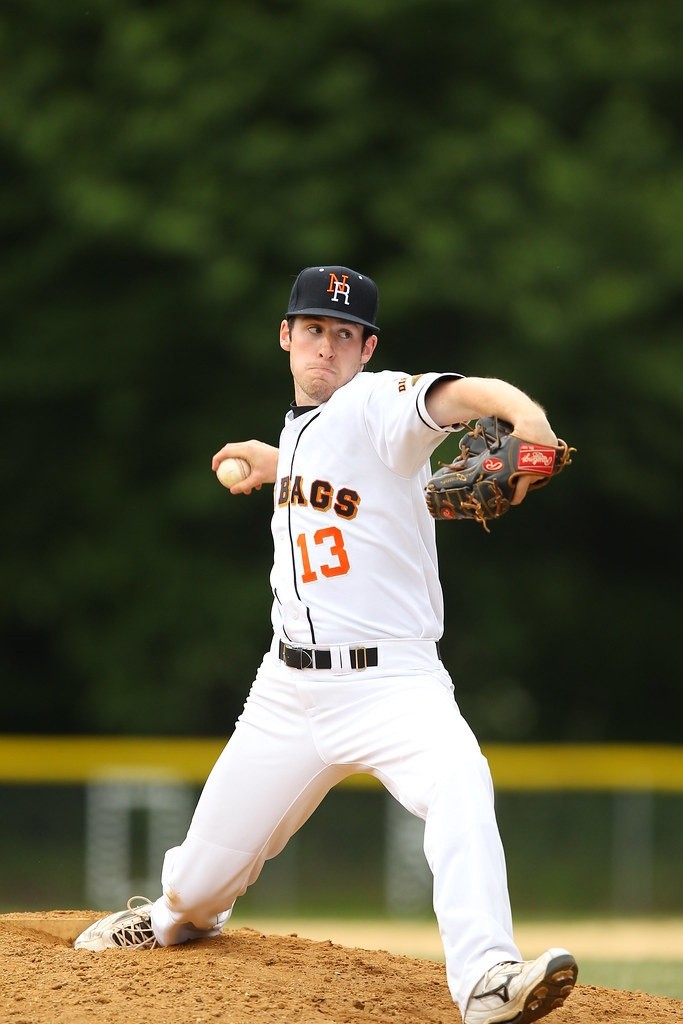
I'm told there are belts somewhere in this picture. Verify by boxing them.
[279,641,378,670]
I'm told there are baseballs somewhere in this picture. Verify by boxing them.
[216,457,251,488]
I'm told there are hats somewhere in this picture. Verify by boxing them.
[285,266,380,331]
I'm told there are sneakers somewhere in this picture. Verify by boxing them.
[464,948,579,1024]
[73,896,162,952]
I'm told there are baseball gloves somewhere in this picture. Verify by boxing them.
[420,397,578,529]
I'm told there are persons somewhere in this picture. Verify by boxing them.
[75,266,580,1023]
